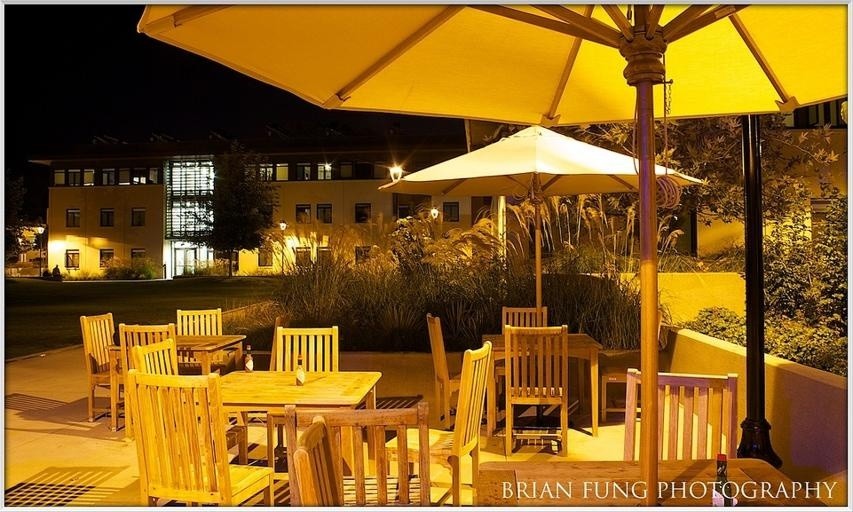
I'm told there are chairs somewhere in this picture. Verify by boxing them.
[78,305,738,507]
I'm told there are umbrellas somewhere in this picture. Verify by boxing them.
[377,124,711,428]
[135,4,848,507]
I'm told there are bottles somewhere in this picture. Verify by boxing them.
[711,453,734,507]
[244,344,254,373]
[294,355,305,386]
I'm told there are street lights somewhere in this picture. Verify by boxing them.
[35,223,44,277]
[388,162,401,229]
[278,219,287,275]
[429,208,438,241]
